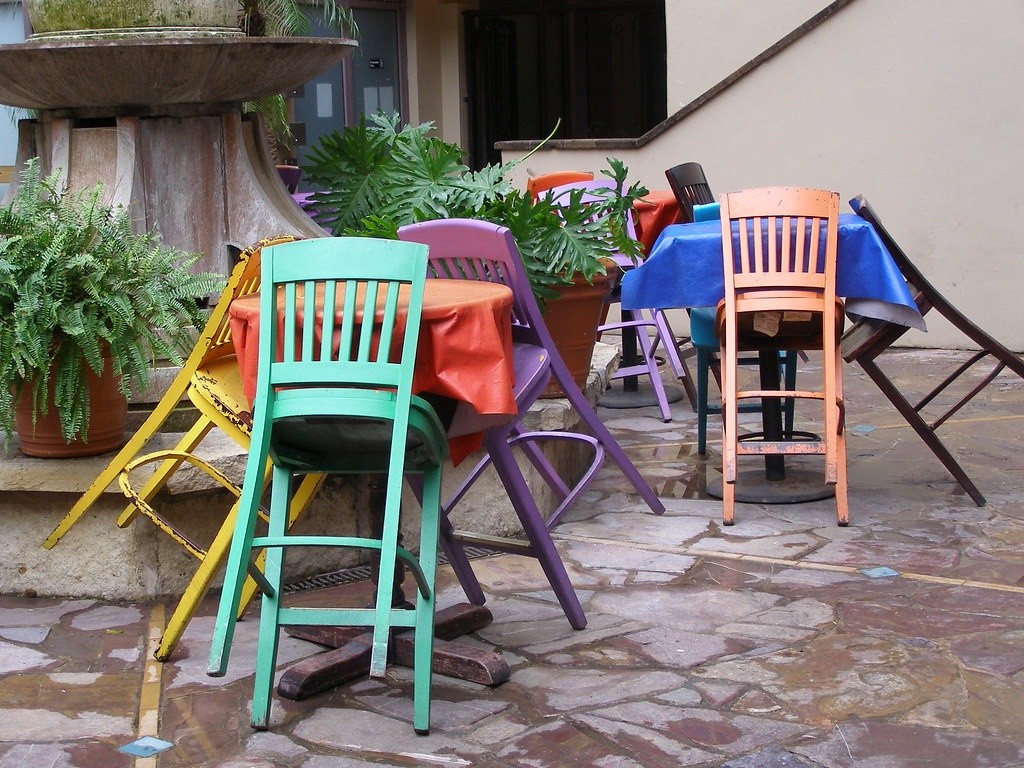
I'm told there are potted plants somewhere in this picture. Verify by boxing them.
[318,111,648,398]
[0,146,226,459]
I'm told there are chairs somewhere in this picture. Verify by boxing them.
[41,163,1024,739]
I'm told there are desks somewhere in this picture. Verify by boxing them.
[618,213,930,505]
[227,278,519,703]
[596,190,694,409]
